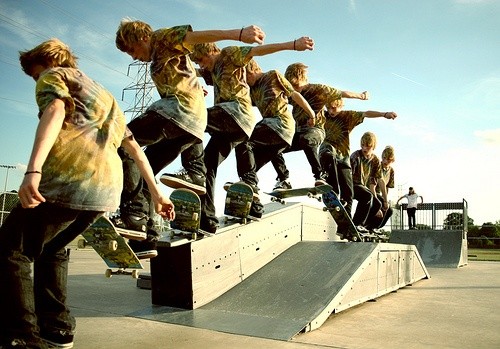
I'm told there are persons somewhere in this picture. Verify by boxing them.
[348,131,390,236]
[270,63,370,188]
[106,17,266,240]
[367,145,396,237]
[158,35,314,237]
[224,57,317,221]
[0,37,178,349]
[314,98,397,238]
[397,183,424,229]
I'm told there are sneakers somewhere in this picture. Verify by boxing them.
[160,169,207,196]
[224,181,259,202]
[314,178,329,187]
[198,218,217,237]
[128,236,158,259]
[40,328,74,348]
[108,210,149,241]
[372,228,383,235]
[355,225,370,235]
[247,204,263,221]
[273,181,292,190]
[336,227,342,236]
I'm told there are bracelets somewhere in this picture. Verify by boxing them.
[239,28,244,41]
[294,39,297,50]
[24,171,44,176]
[363,92,367,100]
[384,112,388,117]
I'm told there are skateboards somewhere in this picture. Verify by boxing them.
[223,182,254,224]
[263,184,333,205]
[167,187,202,240]
[360,232,377,242]
[322,190,363,241]
[378,234,390,243]
[78,215,144,279]
